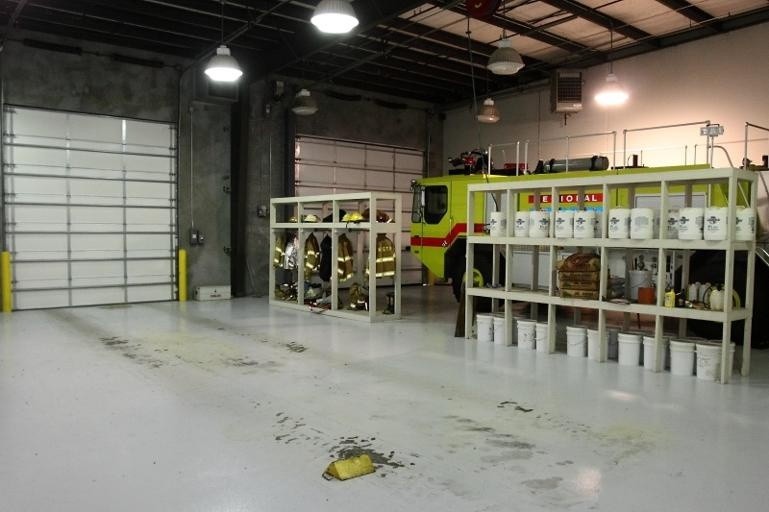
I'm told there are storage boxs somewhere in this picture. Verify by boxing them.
[194,283,233,301]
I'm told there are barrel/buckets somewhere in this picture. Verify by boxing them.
[475,311,549,353]
[606,325,622,360]
[627,269,653,301]
[682,336,707,374]
[575,321,597,357]
[642,335,668,373]
[565,324,586,358]
[668,338,694,376]
[586,327,610,364]
[707,339,736,377]
[653,331,677,369]
[489,206,757,242]
[616,331,641,367]
[627,329,652,366]
[694,343,720,381]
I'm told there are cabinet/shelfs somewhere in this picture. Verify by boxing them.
[463,168,758,386]
[267,191,403,325]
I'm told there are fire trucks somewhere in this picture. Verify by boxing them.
[408,139,767,346]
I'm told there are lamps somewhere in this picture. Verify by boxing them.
[484,2,528,76]
[289,53,316,118]
[591,23,637,109]
[308,2,364,36]
[474,69,503,126]
[204,1,247,84]
